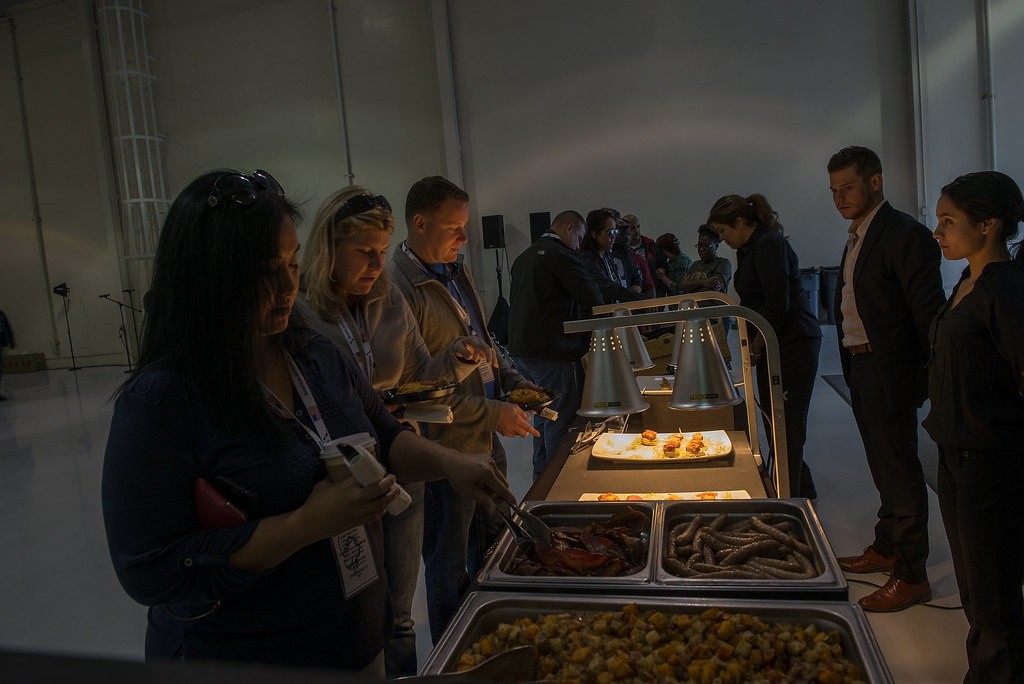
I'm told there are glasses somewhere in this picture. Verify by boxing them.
[695,242,718,248]
[601,228,620,237]
[328,195,392,228]
[192,168,285,230]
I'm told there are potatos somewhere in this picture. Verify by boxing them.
[454,603,865,684]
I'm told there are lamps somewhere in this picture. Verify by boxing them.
[564,291,789,499]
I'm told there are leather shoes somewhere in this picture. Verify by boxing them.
[858,575,931,613]
[837,545,896,574]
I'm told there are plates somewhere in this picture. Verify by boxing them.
[499,386,564,411]
[379,381,458,405]
[635,375,674,394]
[591,430,733,464]
[578,490,752,501]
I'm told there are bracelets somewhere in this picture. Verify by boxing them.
[669,282,677,290]
[749,343,762,357]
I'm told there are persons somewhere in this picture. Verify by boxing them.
[707,193,824,500]
[678,223,732,337]
[826,145,948,613]
[921,170,1024,684]
[574,208,668,332]
[298,185,500,678]
[100,168,517,684]
[385,175,540,648]
[655,233,693,328]
[508,209,609,485]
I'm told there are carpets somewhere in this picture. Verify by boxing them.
[821,375,938,494]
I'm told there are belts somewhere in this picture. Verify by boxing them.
[848,343,873,354]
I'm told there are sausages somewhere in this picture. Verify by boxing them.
[665,514,817,578]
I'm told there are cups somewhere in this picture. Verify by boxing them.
[320,432,377,482]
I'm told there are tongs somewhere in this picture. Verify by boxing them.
[570,421,607,454]
[479,484,553,559]
